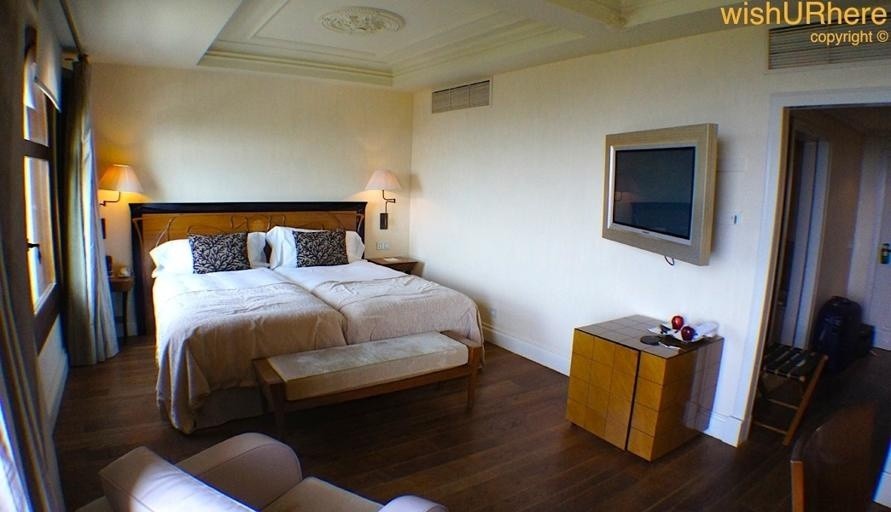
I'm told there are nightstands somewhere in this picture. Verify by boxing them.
[365,257,418,274]
[109,275,134,345]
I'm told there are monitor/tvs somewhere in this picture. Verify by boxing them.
[601,122,718,267]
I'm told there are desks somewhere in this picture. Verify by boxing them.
[566,315,725,461]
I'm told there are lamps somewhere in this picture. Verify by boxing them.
[97,164,143,206]
[365,169,403,229]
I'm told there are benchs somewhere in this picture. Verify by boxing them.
[252,330,483,427]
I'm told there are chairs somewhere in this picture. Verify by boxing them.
[790,400,891,510]
[71,432,450,512]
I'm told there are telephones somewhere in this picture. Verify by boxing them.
[117,267,130,277]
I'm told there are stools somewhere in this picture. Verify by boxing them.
[752,340,828,446]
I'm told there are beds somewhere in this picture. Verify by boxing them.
[129,202,485,433]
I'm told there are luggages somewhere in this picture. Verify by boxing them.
[812,296,862,372]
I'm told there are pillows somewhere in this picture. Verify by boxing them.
[149,225,365,275]
[98,447,257,511]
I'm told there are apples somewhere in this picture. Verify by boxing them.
[681,326,694,341]
[672,316,683,330]
